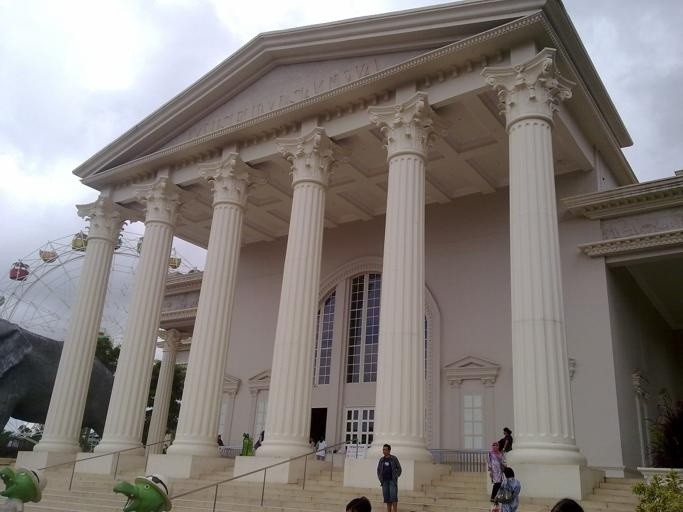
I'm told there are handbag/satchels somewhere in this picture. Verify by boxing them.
[495,487,513,503]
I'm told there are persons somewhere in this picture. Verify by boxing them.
[498,426,512,454]
[376,444,402,511]
[315,435,327,459]
[487,441,506,503]
[309,437,316,446]
[489,468,520,512]
[550,497,585,511]
[345,496,372,512]
[217,434,223,446]
